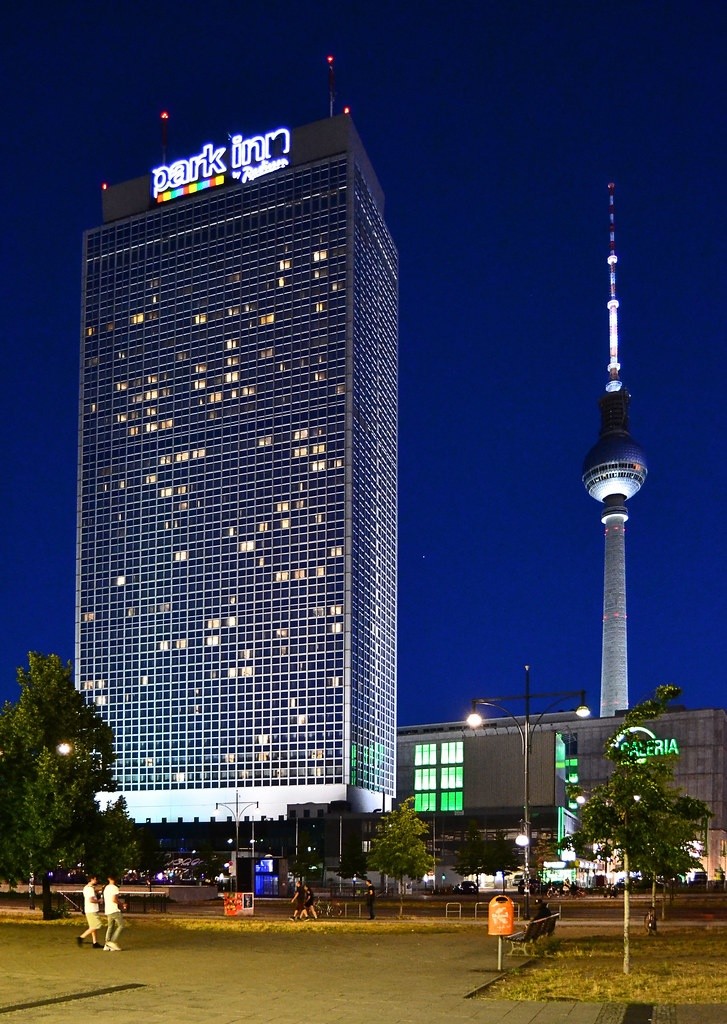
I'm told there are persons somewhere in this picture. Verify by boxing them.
[366,880,376,920]
[103,875,127,951]
[530,898,551,922]
[289,881,319,921]
[434,884,460,894]
[547,884,559,898]
[76,876,103,948]
[570,882,578,896]
[604,885,619,898]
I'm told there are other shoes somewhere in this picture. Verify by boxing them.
[93,942,104,948]
[304,917,310,921]
[76,937,84,947]
[107,942,122,951]
[103,944,114,951]
[289,917,295,922]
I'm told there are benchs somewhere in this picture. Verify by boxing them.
[504,913,560,957]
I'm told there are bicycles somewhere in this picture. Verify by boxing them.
[308,896,343,918]
[643,900,660,936]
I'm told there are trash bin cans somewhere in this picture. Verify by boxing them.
[117,897,130,913]
[487,895,515,935]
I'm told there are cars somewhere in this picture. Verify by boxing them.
[518,878,547,893]
[687,872,706,885]
[459,881,478,893]
[547,885,570,898]
[512,874,525,886]
[73,889,130,912]
[611,882,637,894]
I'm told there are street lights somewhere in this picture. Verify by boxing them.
[467,664,590,921]
[577,776,642,873]
[215,790,260,892]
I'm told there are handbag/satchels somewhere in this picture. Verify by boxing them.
[100,884,108,905]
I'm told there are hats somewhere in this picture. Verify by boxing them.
[534,899,542,905]
